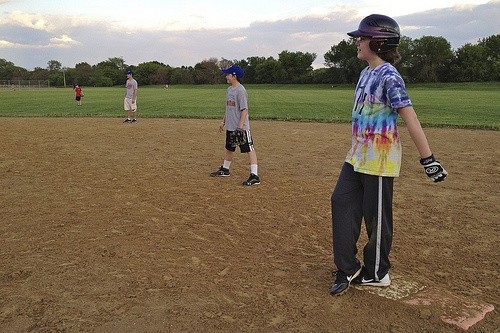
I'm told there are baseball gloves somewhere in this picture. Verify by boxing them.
[229,127,247,147]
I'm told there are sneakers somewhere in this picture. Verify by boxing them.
[210,166,230,177]
[330,264,361,296]
[352,265,390,286]
[123,119,131,123]
[243,173,260,185]
[130,118,136,123]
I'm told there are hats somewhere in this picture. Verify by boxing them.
[126,70,134,75]
[222,64,244,79]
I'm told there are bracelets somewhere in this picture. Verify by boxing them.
[221,121,225,125]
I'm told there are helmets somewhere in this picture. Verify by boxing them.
[347,13,400,53]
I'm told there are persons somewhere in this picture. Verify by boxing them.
[330,14,449,298]
[123,70,138,123]
[75,84,82,106]
[209,64,260,187]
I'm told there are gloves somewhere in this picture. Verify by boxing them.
[419,153,448,183]
